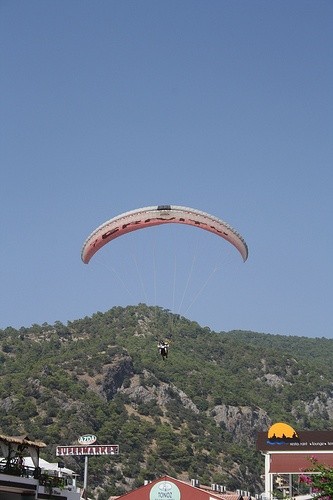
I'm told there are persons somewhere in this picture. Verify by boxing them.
[159,339,168,361]
